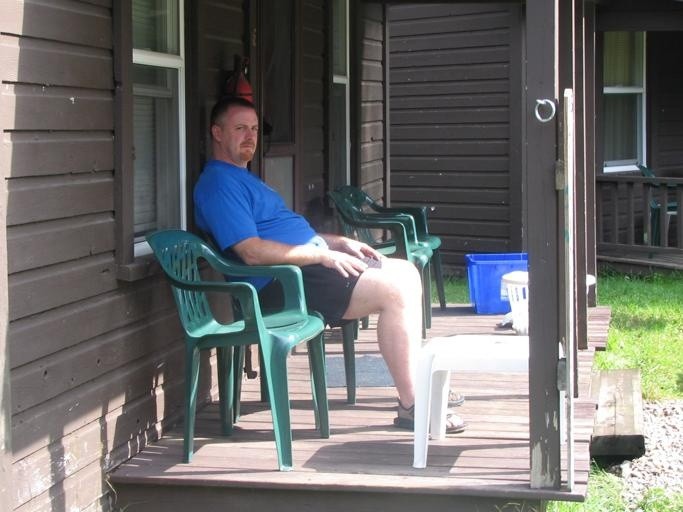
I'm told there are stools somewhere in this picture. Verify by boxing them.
[411,333,568,470]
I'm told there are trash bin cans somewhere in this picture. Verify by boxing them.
[501,271,530,335]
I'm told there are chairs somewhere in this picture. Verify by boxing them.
[190,215,362,432]
[147,229,334,472]
[335,185,446,325]
[634,162,682,247]
[327,189,437,338]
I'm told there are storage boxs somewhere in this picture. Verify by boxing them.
[465,252,528,316]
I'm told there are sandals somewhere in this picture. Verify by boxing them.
[392,386,468,433]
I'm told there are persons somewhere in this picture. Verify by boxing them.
[191,95,468,434]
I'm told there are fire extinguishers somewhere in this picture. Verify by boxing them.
[224,55,251,102]
